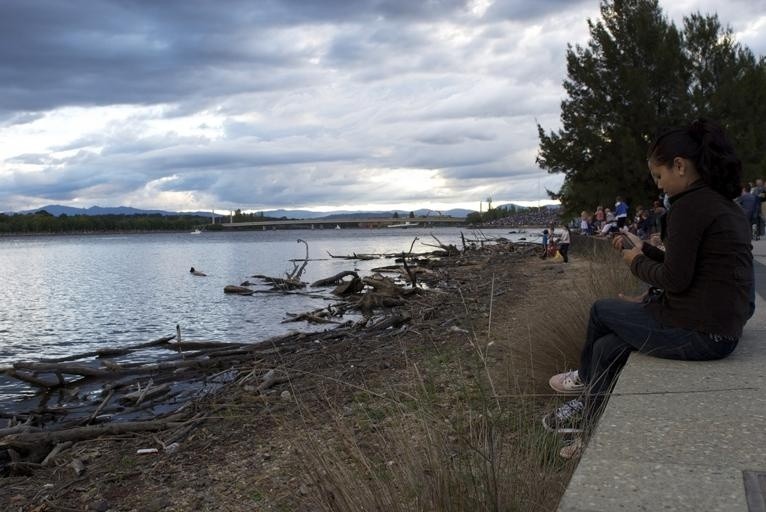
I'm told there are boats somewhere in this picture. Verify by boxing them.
[388,222,419,228]
[191,229,202,234]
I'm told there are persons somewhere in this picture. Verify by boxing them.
[542,134,754,432]
[544,224,572,264]
[580,194,669,238]
[736,179,765,241]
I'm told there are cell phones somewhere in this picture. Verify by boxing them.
[615,233,636,249]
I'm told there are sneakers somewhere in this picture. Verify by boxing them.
[549,371,585,394]
[560,434,577,444]
[544,400,588,433]
[560,444,584,459]
